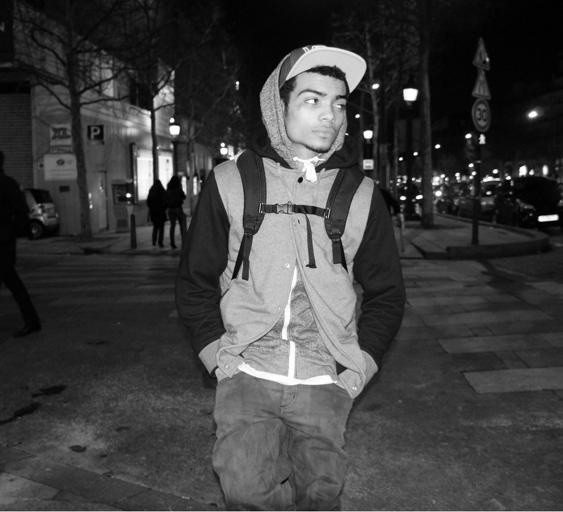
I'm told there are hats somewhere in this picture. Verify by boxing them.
[278,45,367,95]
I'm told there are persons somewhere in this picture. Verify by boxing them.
[0,152,42,339]
[145,180,166,248]
[164,175,187,250]
[168,41,408,509]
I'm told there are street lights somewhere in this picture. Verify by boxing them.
[167,120,181,180]
[219,140,227,163]
[399,80,419,222]
[360,127,373,179]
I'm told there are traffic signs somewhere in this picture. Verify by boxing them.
[471,97,492,132]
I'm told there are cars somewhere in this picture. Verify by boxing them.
[395,174,562,234]
[20,187,62,241]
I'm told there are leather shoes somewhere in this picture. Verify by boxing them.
[14,318,43,337]
